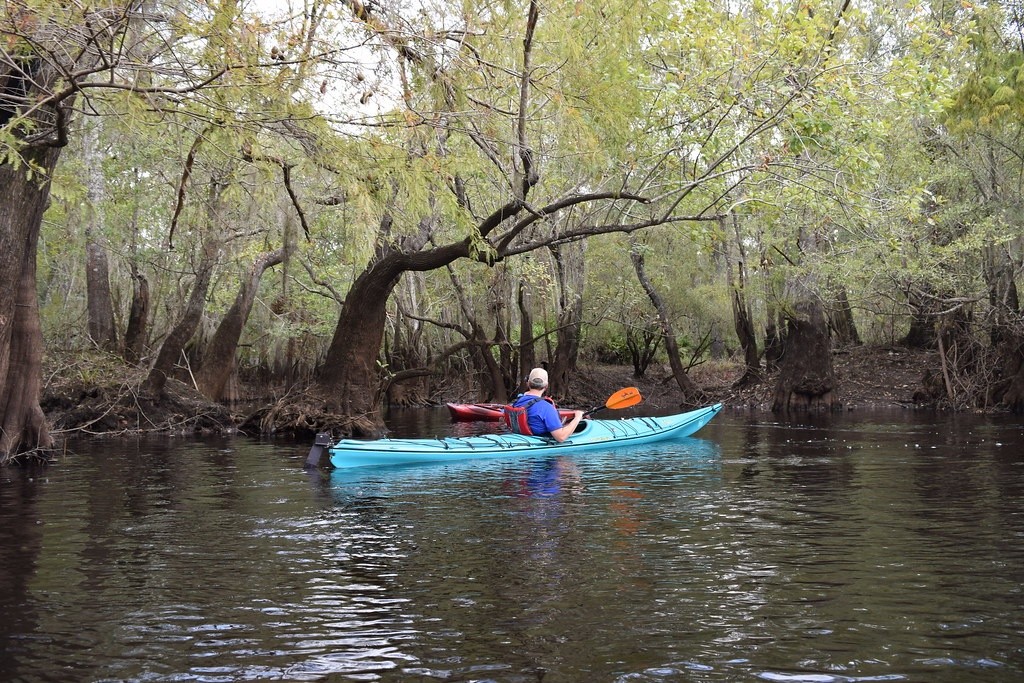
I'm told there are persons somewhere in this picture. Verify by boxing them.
[509,368,584,443]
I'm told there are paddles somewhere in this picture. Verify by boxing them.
[566,386,642,424]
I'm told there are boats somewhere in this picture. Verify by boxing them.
[306,400,727,469]
[447,401,505,423]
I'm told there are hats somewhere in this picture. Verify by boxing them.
[529,367,548,388]
